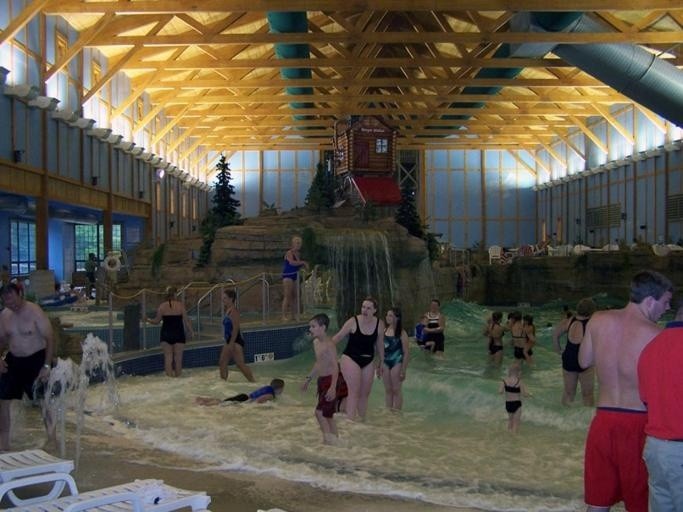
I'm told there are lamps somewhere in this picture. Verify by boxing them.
[155,168,165,181]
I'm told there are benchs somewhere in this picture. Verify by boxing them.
[11,447,71,500]
[1,473,212,512]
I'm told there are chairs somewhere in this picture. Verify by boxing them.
[487,245,502,265]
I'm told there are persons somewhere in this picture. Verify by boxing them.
[424,299,446,358]
[507,312,514,328]
[578,270,673,512]
[219,290,256,382]
[85,253,96,299]
[553,301,595,408]
[143,287,195,377]
[637,303,683,511]
[197,378,285,407]
[415,317,435,353]
[297,313,349,446]
[511,311,533,366]
[0,283,55,453]
[382,307,409,409]
[333,299,384,420]
[488,312,504,365]
[104,251,120,280]
[498,365,528,431]
[524,315,536,363]
[282,237,302,321]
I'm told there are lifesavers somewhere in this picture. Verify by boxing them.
[104,255,121,271]
